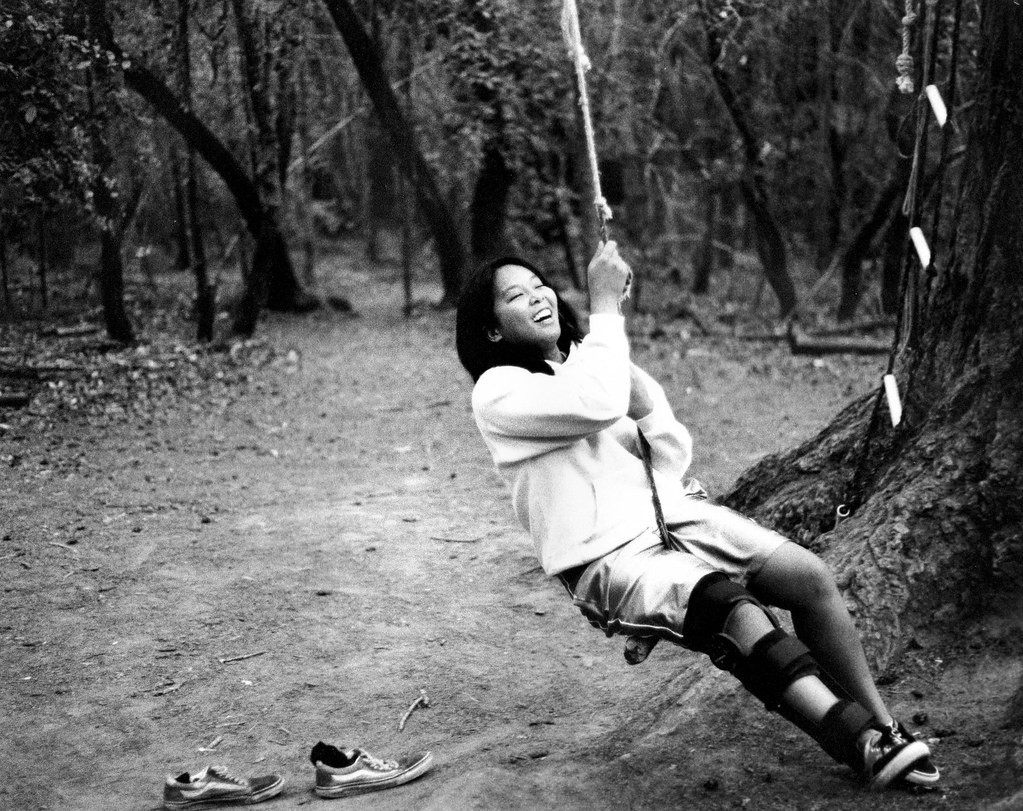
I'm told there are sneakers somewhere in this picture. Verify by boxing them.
[314,745,434,799]
[874,721,941,785]
[865,732,932,793]
[162,762,286,811]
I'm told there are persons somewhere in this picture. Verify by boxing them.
[455,241,941,789]
